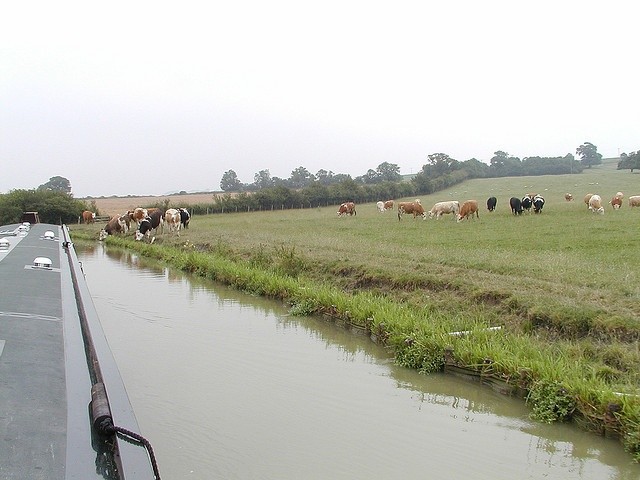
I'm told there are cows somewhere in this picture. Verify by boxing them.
[509,196,524,215]
[589,194,605,215]
[376,201,384,213]
[455,200,479,224]
[336,203,347,218]
[532,193,546,214]
[584,192,594,209]
[616,191,623,198]
[384,200,395,210]
[99,214,122,241]
[132,208,163,226]
[428,201,461,221]
[565,192,573,203]
[347,201,357,216]
[135,210,164,241]
[83,211,96,226]
[179,207,190,231]
[113,210,134,234]
[628,195,640,210]
[397,201,427,222]
[522,193,532,212]
[165,208,182,238]
[611,197,623,210]
[487,196,497,212]
[415,198,421,203]
